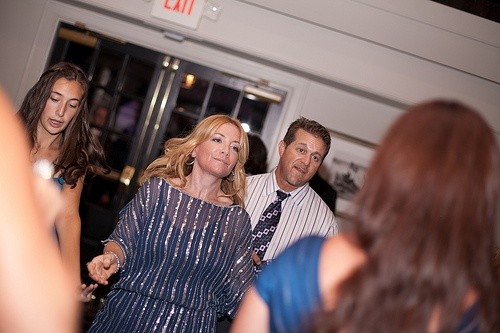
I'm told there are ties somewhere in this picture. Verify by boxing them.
[252,189,291,279]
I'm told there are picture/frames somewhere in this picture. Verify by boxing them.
[320,138,377,218]
